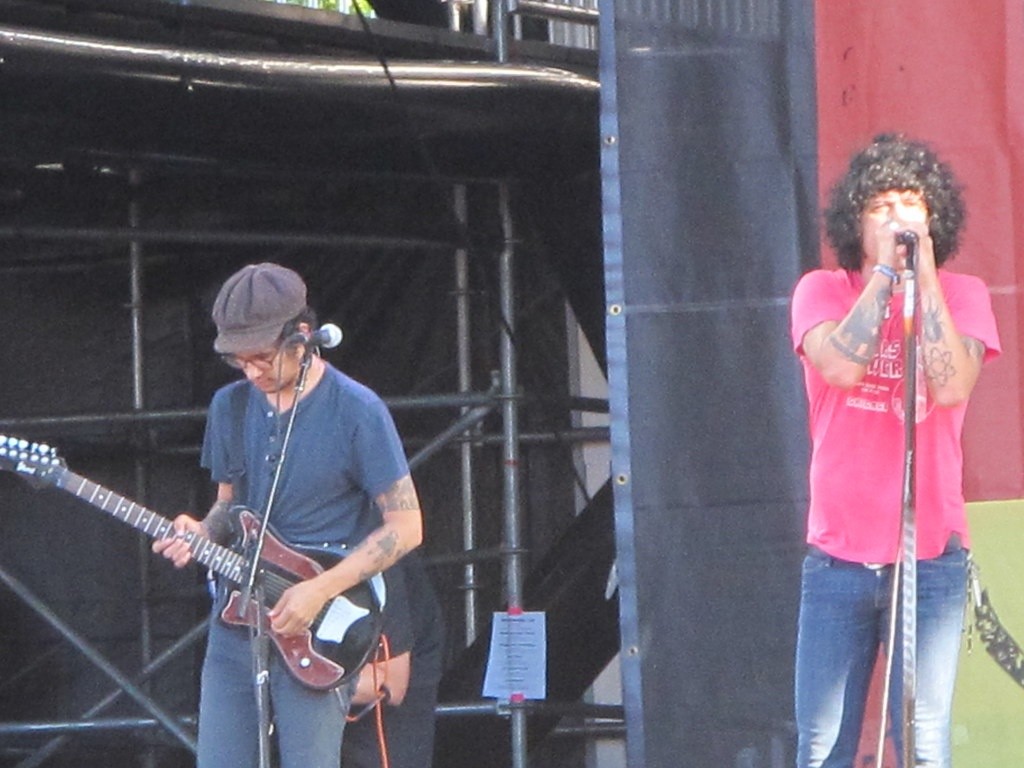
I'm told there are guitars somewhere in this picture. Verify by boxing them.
[0,432,389,694]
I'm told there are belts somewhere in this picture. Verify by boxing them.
[808,532,965,569]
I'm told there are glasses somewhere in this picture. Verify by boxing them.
[220,340,283,370]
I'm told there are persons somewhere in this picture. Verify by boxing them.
[791,134,1002,768]
[339,550,443,768]
[152,263,423,768]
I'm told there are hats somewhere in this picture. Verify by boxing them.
[212,263,307,353]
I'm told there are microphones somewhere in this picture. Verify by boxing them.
[289,323,342,349]
[895,232,920,248]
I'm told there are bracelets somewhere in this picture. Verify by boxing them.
[872,264,901,286]
